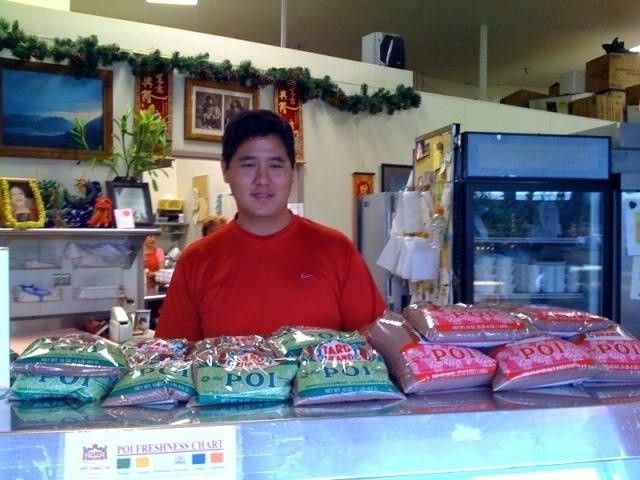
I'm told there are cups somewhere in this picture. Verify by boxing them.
[135,308,150,331]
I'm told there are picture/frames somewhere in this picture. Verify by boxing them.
[0,57,114,161]
[108,181,155,227]
[0,177,46,229]
[184,78,260,143]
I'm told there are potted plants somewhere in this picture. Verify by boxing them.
[68,106,173,205]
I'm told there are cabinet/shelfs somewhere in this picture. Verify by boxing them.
[1,229,160,319]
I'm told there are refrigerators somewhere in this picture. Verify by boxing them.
[453,131,615,320]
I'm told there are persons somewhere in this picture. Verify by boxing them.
[198,95,220,129]
[147,109,386,360]
[8,183,39,223]
[224,97,246,128]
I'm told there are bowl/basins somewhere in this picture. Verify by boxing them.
[474,249,584,298]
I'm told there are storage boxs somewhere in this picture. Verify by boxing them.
[499,52,640,126]
